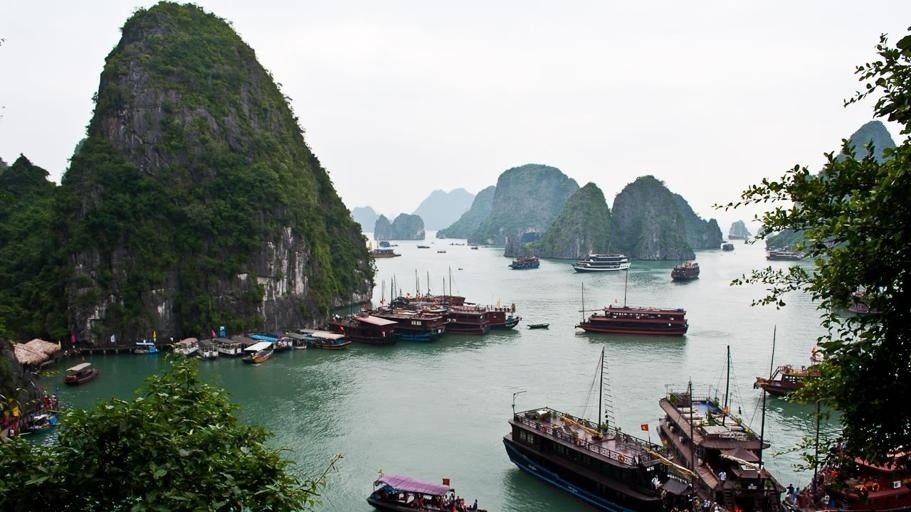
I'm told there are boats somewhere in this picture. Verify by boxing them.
[26,413,57,434]
[509,252,541,272]
[370,248,402,259]
[526,322,551,329]
[722,243,736,253]
[59,361,98,385]
[571,249,634,275]
[417,239,481,255]
[762,249,810,260]
[378,240,398,248]
[569,267,694,336]
[672,257,702,280]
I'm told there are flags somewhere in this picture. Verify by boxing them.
[722,405,729,414]
[641,424,649,431]
[443,478,450,485]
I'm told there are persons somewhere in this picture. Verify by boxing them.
[787,442,862,512]
[379,489,480,511]
[650,467,728,512]
[0,385,58,430]
